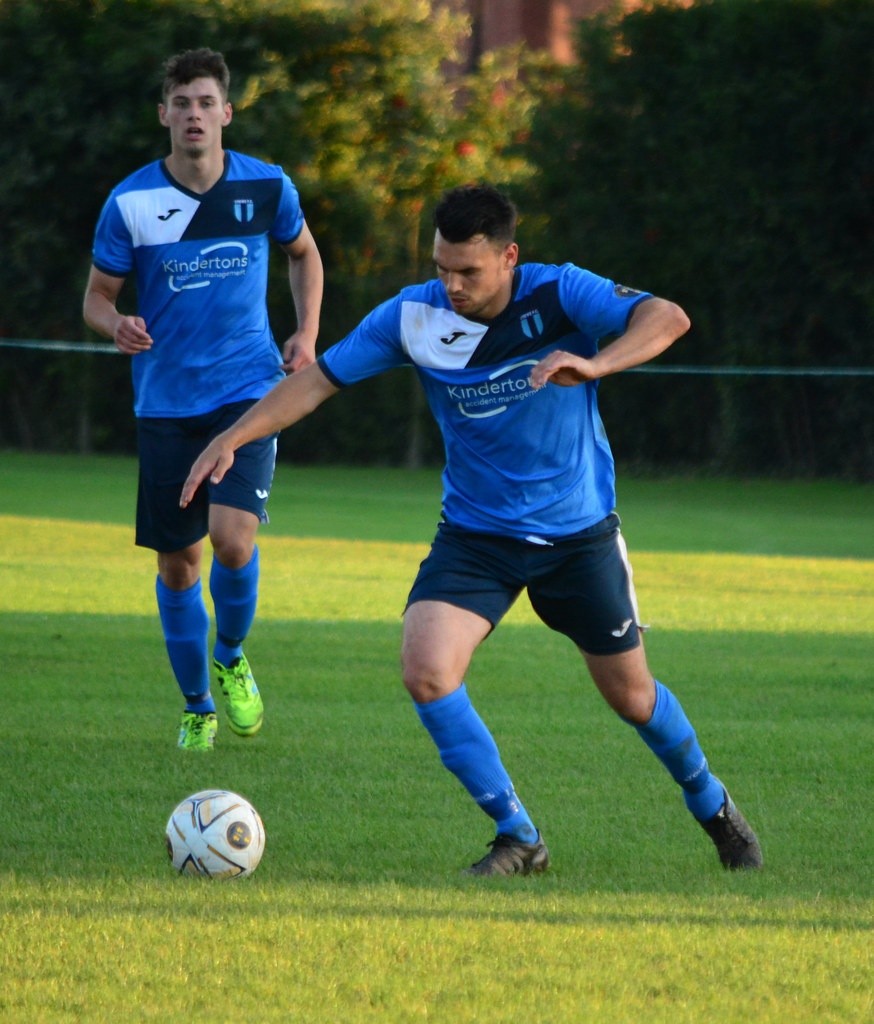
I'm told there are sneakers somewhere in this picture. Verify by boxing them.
[693,776,764,870]
[471,829,549,875]
[211,653,266,736]
[177,710,219,751]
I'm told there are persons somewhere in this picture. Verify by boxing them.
[81,42,324,748]
[179,184,763,886]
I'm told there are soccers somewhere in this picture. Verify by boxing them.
[165,790,266,880]
